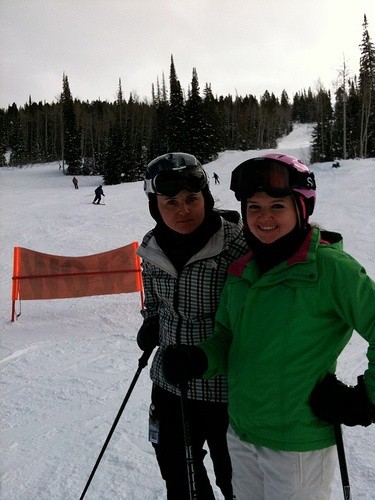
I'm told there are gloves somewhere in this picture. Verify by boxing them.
[136,316,160,350]
[161,343,208,385]
[308,372,375,427]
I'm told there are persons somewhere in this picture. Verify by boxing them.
[212,173,220,185]
[72,177,79,189]
[137,152,253,500]
[162,154,374,500]
[93,185,105,204]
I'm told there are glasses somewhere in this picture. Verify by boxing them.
[230,157,316,198]
[143,169,207,197]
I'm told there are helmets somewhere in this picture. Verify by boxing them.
[145,153,205,180]
[262,153,316,215]
